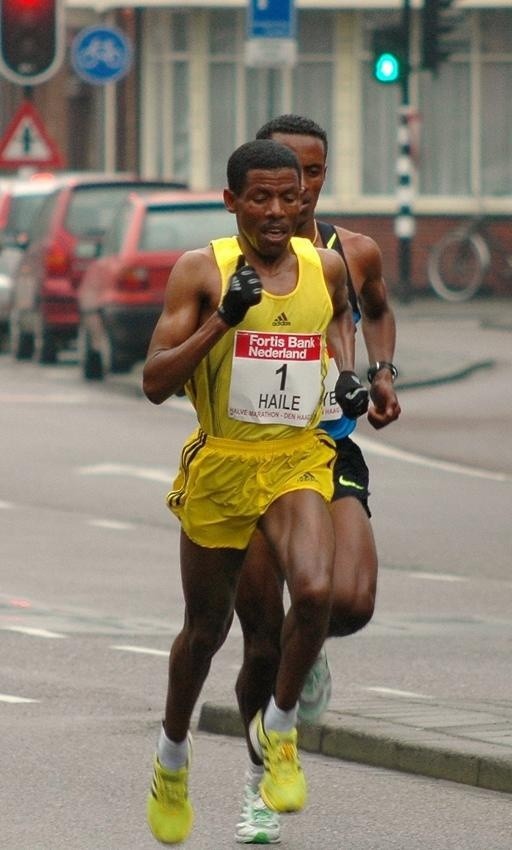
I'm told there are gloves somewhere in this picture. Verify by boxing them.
[335,369,369,420]
[218,254,263,328]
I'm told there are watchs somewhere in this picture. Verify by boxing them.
[365,361,399,380]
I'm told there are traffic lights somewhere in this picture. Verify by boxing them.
[372,23,410,84]
[3,0,67,87]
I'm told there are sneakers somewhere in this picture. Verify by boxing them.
[298,646,331,723]
[235,771,282,845]
[249,708,307,815]
[148,731,193,845]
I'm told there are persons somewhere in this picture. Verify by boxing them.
[143,139,369,846]
[175,116,401,845]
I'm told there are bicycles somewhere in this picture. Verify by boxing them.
[425,209,511,301]
